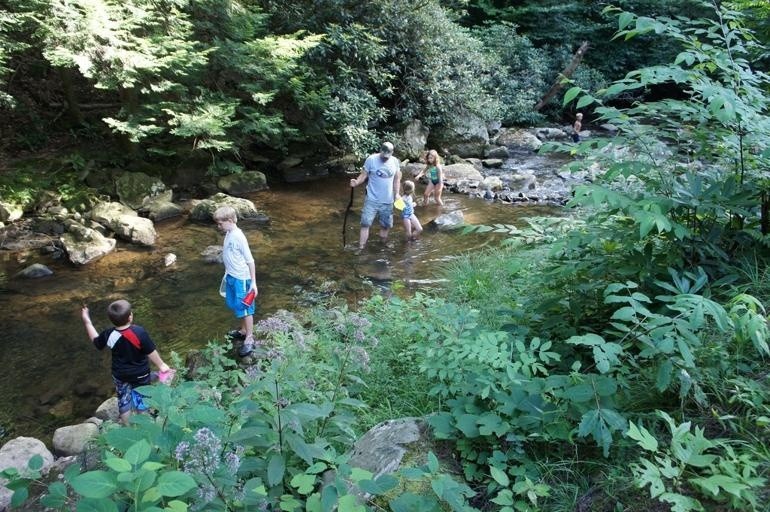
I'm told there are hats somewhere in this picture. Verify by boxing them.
[382,142,393,158]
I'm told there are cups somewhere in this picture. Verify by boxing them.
[241,291,256,308]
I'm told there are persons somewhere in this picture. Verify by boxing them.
[570,112,584,143]
[396,179,423,244]
[349,142,401,255]
[81,300,171,429]
[201,206,258,358]
[414,149,444,207]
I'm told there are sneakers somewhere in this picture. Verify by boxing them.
[239,338,255,356]
[227,328,246,340]
